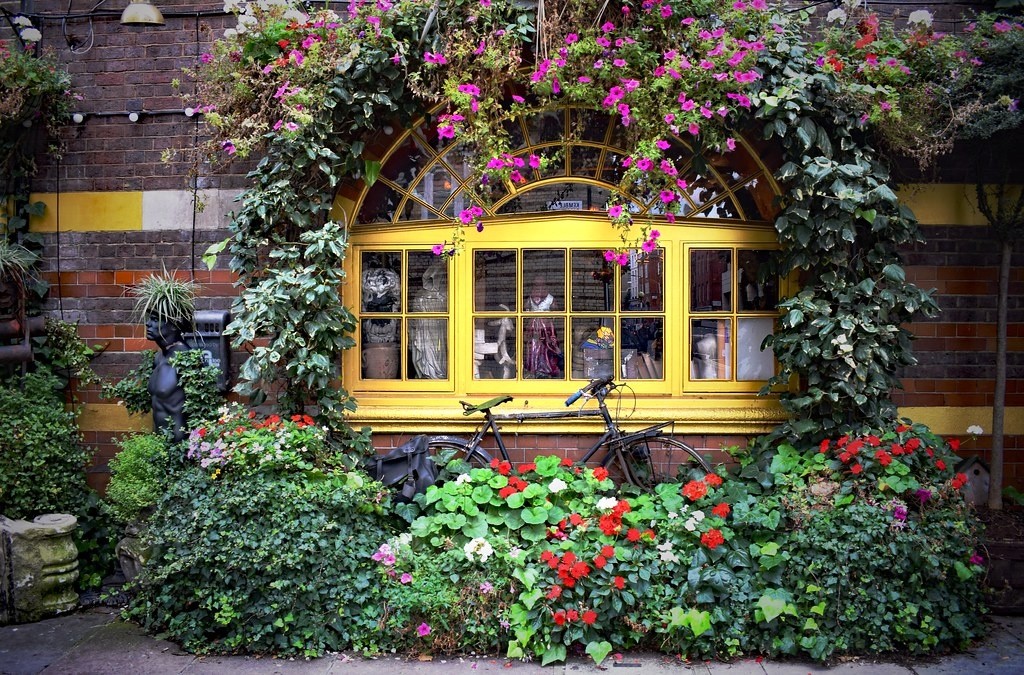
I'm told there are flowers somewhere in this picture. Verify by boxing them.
[596,326,614,349]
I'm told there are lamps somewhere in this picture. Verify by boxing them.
[119,0,166,28]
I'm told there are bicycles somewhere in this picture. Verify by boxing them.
[428,374,711,495]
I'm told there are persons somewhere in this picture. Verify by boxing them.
[525,274,564,378]
[145,303,201,449]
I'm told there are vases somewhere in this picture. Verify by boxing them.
[361,342,398,379]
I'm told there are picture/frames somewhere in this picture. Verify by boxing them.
[582,349,637,379]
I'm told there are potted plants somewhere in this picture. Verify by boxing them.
[958,128,1023,616]
